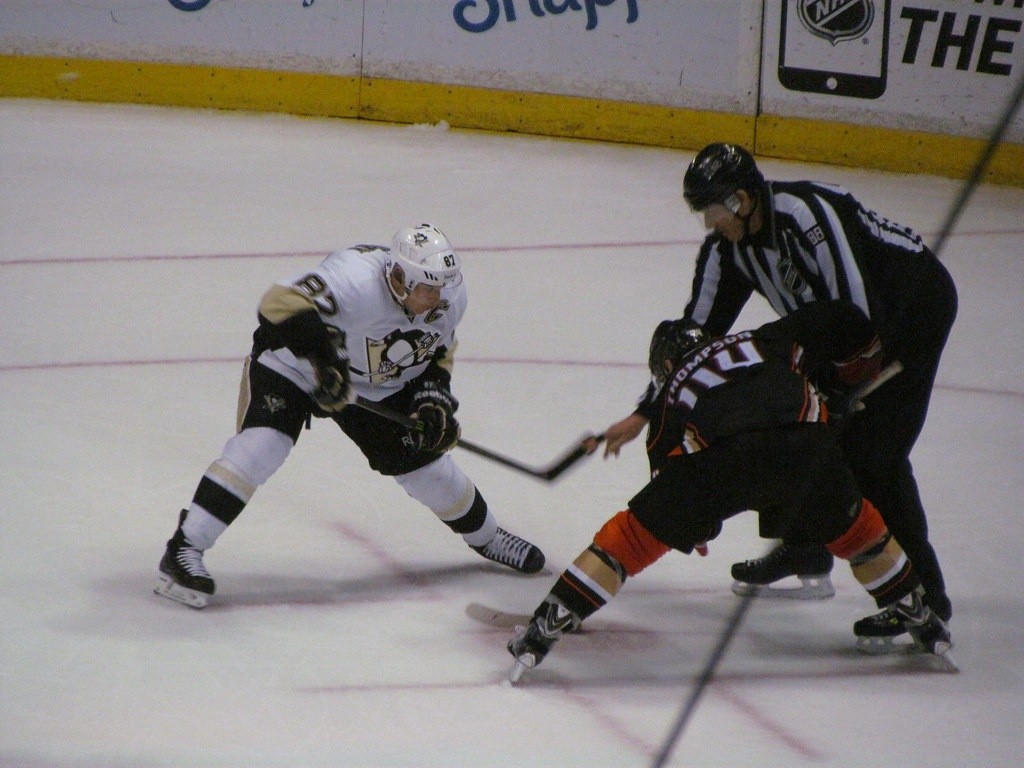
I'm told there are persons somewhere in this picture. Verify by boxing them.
[589,143,958,653]
[155,223,554,607]
[508,300,959,685]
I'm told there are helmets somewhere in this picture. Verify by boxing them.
[385,223,463,291]
[648,318,714,383]
[682,142,758,212]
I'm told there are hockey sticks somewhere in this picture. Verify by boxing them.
[346,390,595,482]
[466,358,906,629]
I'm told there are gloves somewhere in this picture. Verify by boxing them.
[406,381,462,457]
[832,335,897,406]
[307,341,354,415]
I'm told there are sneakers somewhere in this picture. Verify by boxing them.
[731,539,837,600]
[853,584,953,654]
[468,526,545,574]
[151,508,215,608]
[507,595,582,686]
[894,591,960,674]
